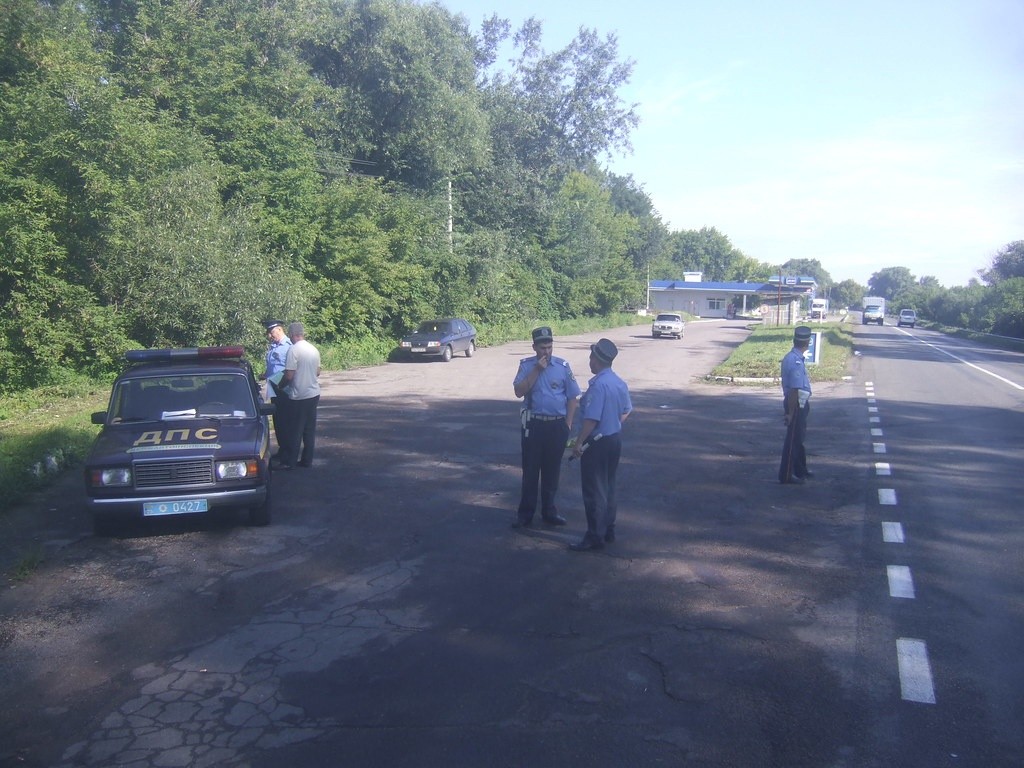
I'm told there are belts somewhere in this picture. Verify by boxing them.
[531,414,563,421]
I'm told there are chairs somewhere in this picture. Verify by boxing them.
[135,384,174,417]
[204,380,240,405]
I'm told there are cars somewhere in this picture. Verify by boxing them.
[651,314,685,339]
[84,348,279,529]
[399,318,477,363]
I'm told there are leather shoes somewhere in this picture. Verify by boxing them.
[512,518,526,527]
[543,515,567,525]
[569,540,605,550]
[606,537,614,541]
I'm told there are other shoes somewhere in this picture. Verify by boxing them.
[297,462,312,467]
[272,463,295,471]
[803,471,812,476]
[272,453,279,459]
[790,476,803,484]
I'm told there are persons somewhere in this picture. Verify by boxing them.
[569,338,632,552]
[273,323,320,472]
[779,326,814,484]
[258,319,293,460]
[512,326,581,528]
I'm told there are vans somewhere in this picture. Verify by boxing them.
[898,309,915,328]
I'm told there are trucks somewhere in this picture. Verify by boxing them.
[862,297,886,325]
[810,299,828,319]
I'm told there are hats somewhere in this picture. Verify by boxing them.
[794,326,812,341]
[532,326,553,343]
[261,320,284,332]
[590,338,618,363]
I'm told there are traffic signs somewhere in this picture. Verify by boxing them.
[803,332,821,364]
